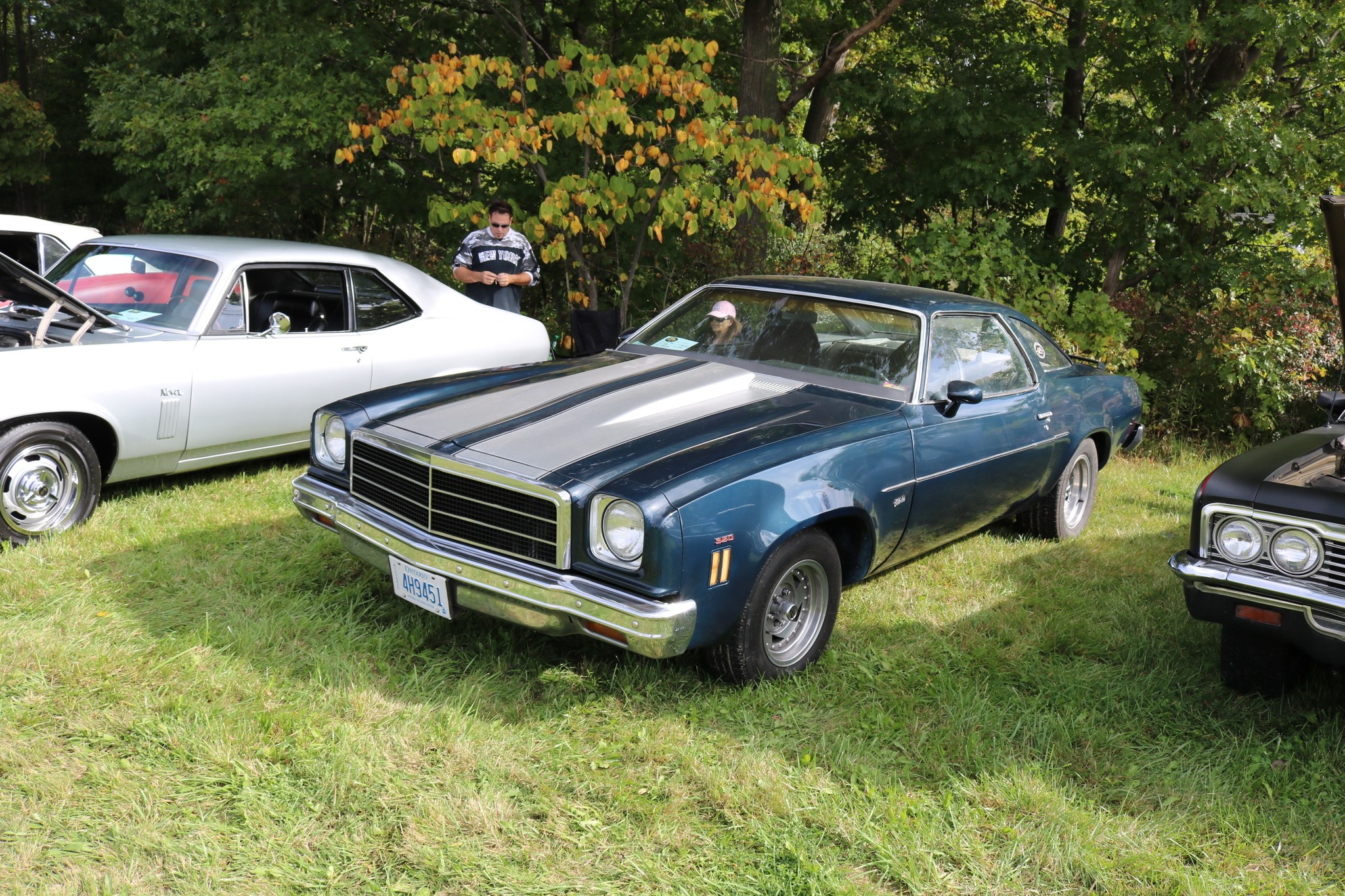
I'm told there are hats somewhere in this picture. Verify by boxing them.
[704,300,736,318]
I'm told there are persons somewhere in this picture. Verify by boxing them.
[686,300,747,359]
[449,201,541,314]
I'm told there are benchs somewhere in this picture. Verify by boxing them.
[248,287,346,335]
[824,336,998,402]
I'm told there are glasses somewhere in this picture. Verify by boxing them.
[708,316,731,322]
[490,216,509,228]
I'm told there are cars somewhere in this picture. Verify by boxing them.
[1166,197,1345,696]
[293,276,1147,691]
[0,214,551,543]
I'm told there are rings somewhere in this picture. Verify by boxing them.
[501,282,504,286]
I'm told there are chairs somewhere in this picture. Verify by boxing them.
[249,303,276,335]
[171,279,216,327]
[883,324,942,396]
[752,304,818,365]
